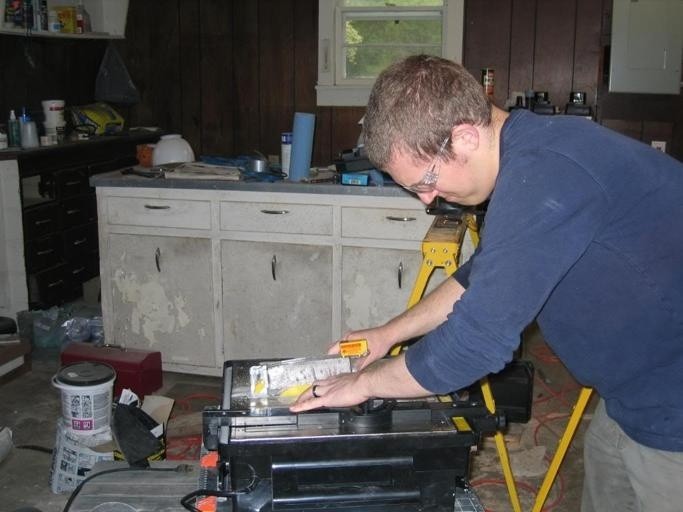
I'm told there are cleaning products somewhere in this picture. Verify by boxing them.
[20,108,32,124]
[8,110,21,148]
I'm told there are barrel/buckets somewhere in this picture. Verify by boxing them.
[50,361,117,432]
[41,100,65,121]
[43,121,67,143]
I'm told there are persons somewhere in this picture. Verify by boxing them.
[288,54,683,512]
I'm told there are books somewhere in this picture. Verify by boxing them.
[5,0,77,33]
[165,163,242,180]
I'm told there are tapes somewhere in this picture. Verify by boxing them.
[247,156,271,172]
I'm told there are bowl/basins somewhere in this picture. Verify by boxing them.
[151,133,196,167]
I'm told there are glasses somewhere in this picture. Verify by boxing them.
[401,120,474,194]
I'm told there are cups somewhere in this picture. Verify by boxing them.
[285,112,318,184]
[136,143,154,168]
[40,99,67,146]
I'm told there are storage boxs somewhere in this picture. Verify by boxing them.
[61,343,163,400]
[0,336,32,387]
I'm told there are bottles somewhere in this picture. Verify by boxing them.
[3,0,95,33]
[5,105,40,150]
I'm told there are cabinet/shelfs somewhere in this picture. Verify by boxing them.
[0,131,161,332]
[88,156,476,378]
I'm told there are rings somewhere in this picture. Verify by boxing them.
[312,385,320,399]
